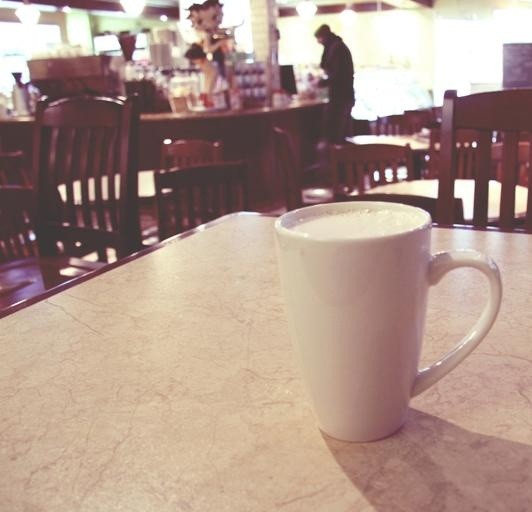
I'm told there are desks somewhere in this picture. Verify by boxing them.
[1,207,530,512]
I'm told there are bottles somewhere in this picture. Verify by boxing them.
[11,72,33,113]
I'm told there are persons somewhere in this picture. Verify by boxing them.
[308,23,356,176]
[186,0,237,58]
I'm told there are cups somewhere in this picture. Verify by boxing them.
[273,199,502,443]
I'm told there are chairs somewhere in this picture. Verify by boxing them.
[1,89,531,198]
[433,89,532,233]
[1,92,141,315]
[152,159,246,240]
